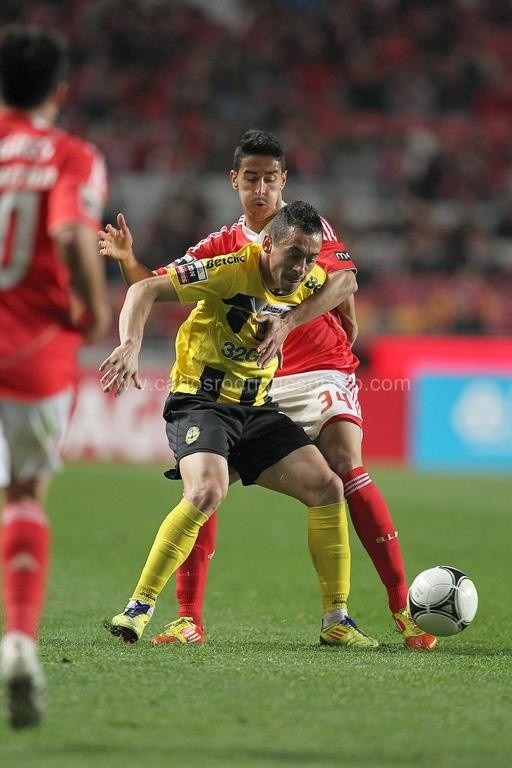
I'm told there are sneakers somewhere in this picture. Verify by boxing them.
[110,600,154,643]
[392,608,437,652]
[2,631,45,730]
[150,617,204,645]
[319,616,379,650]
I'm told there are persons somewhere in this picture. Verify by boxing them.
[100,131,438,650]
[0,28,111,732]
[1,0,511,337]
[100,201,381,650]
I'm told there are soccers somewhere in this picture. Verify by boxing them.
[408,565,478,635]
